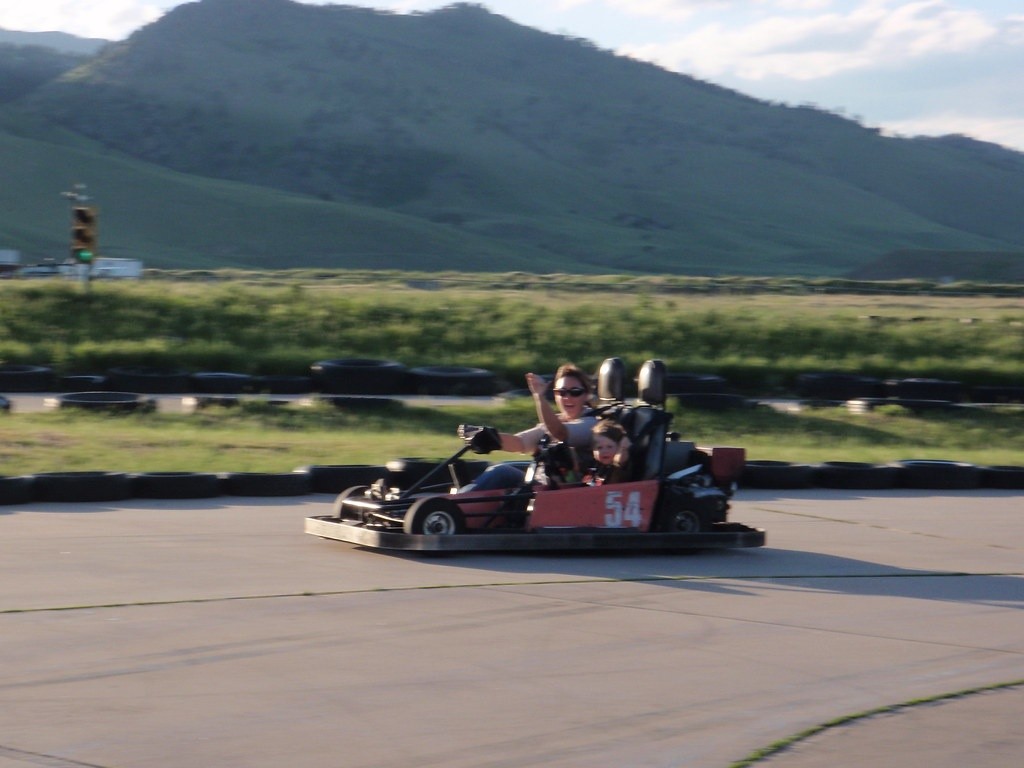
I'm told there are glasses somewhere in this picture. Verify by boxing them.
[552,387,587,398]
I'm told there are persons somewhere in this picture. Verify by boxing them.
[468,364,629,528]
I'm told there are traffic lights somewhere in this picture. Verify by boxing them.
[70,206,95,263]
